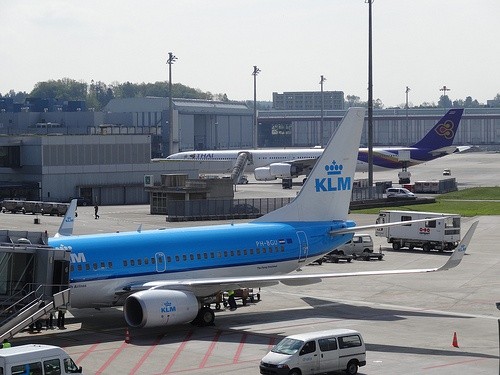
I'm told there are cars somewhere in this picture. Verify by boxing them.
[387,188,417,199]
[443,169,451,176]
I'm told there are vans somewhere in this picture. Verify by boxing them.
[261,328,365,374]
[334,235,372,259]
[0,345,82,375]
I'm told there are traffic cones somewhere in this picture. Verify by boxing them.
[452,333,460,348]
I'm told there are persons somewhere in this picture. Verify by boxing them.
[2,339,11,348]
[46,314,55,329]
[35,319,42,333]
[94,204,99,219]
[228,290,237,310]
[29,323,34,332]
[58,310,66,329]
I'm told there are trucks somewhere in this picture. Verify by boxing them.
[376,208,461,254]
[3,200,78,217]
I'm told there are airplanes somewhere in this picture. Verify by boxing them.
[44,106,481,334]
[166,109,477,188]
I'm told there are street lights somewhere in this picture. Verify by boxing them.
[251,65,261,149]
[440,86,450,114]
[167,51,177,111]
[319,75,328,146]
[405,87,412,147]
